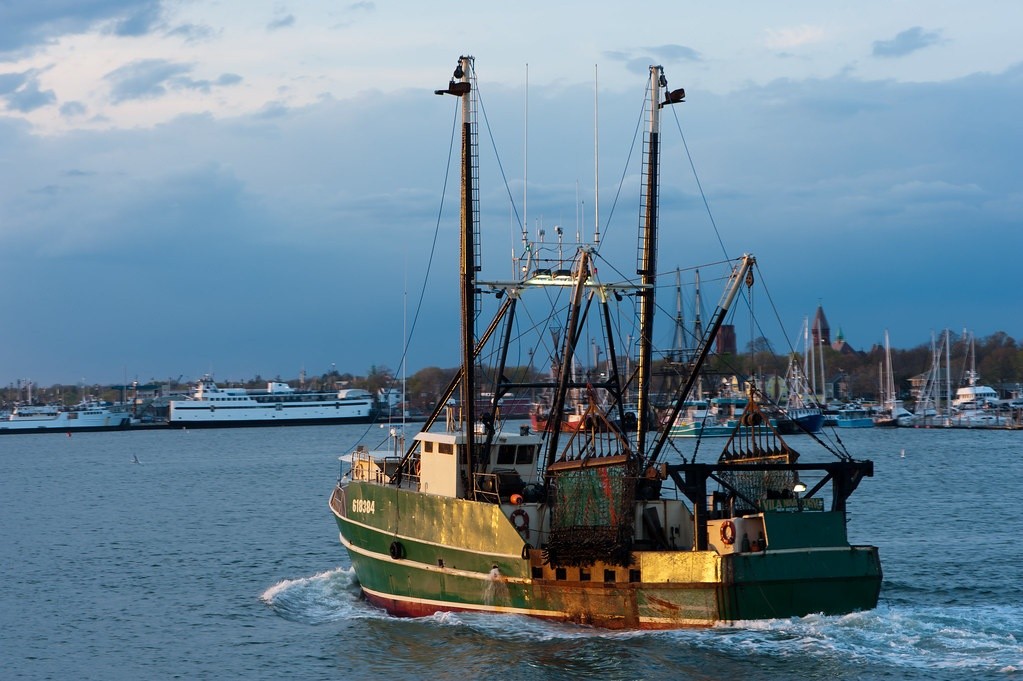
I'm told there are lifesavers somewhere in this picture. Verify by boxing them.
[511,509,529,532]
[720,521,736,544]
[416,462,420,476]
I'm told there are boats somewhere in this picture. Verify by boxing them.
[0,393,139,432]
[166,375,377,424]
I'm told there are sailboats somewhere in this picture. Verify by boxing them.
[326,50,887,629]
[517,259,1023,447]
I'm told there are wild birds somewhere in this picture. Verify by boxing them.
[900,449,908,460]
[130,453,143,465]
